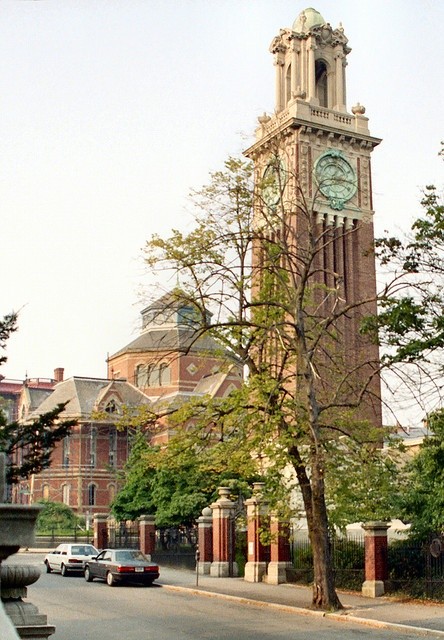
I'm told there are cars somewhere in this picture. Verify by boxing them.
[84,549,159,586]
[44,544,99,577]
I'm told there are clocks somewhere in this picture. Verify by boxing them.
[312,147,359,210]
[259,157,287,218]
